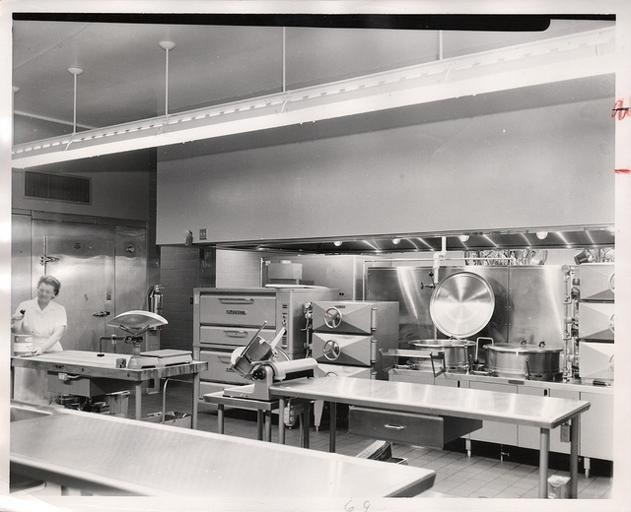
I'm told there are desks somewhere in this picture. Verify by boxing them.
[269,378,590,498]
[11,410,436,498]
[11,349,207,430]
[204,391,310,450]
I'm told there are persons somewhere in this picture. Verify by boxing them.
[11,273,70,406]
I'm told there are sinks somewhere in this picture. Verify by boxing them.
[10,402,55,421]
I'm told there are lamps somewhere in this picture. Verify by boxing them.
[11,25,616,170]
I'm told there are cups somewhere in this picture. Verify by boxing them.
[116,358,126,368]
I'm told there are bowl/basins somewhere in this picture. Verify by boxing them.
[128,356,141,369]
